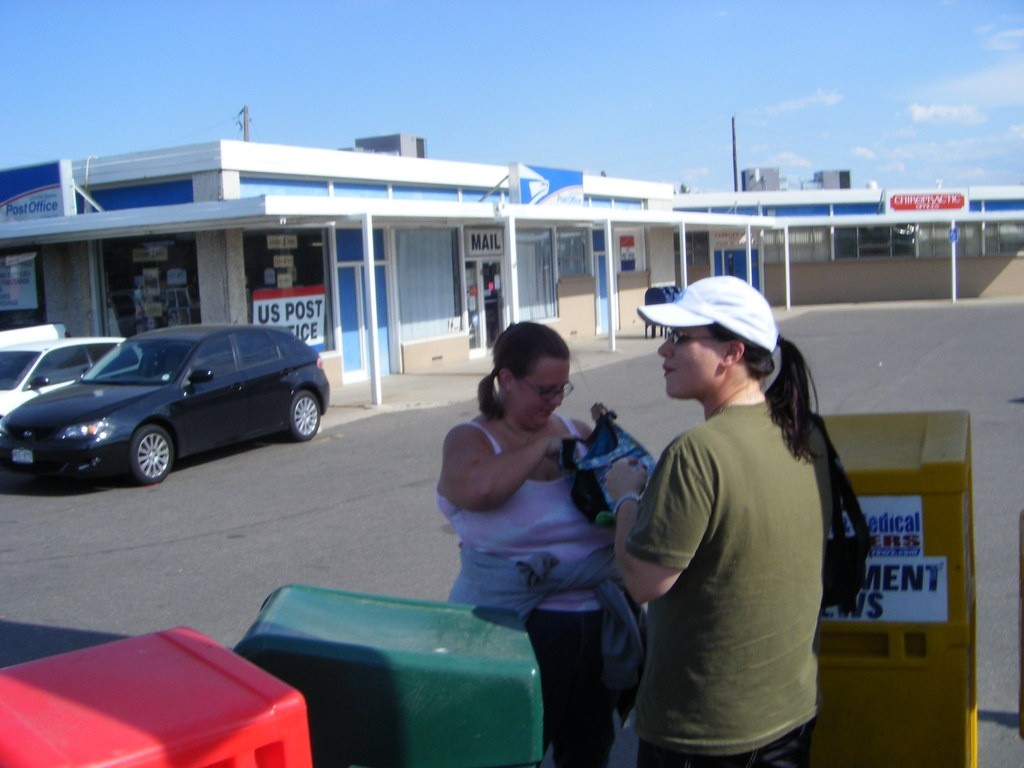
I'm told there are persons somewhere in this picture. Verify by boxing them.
[435,321,645,768]
[602,275,833,768]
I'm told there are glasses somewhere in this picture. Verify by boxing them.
[518,374,573,401]
[667,330,719,345]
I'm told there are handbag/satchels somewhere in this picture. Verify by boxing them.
[812,414,879,610]
[560,410,655,524]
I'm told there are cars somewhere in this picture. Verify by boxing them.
[0,325,330,486]
[0,335,144,421]
[0,321,70,347]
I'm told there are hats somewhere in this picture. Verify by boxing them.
[636,276,779,353]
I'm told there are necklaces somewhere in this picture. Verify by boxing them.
[504,421,538,443]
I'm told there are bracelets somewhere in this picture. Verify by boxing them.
[614,492,640,516]
